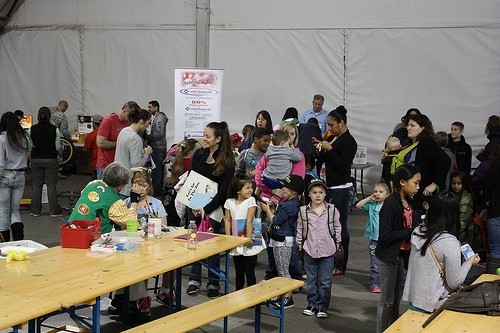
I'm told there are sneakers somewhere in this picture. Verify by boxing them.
[303,306,316,315]
[187,285,199,295]
[369,284,381,293]
[317,309,327,317]
[155,290,172,308]
[275,296,294,309]
[208,289,220,298]
[138,296,151,316]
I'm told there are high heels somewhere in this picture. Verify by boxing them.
[303,272,308,278]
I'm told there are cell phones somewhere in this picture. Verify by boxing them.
[460,244,478,261]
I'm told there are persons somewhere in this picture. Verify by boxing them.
[402,189,480,313]
[259,175,306,308]
[375,164,423,333]
[46,100,72,178]
[356,108,500,293]
[224,171,267,308]
[297,179,341,318]
[184,121,239,298]
[27,106,66,217]
[117,169,180,317]
[12,109,31,138]
[0,112,32,242]
[84,93,358,293]
[66,162,147,319]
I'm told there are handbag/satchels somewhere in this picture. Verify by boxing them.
[422,280,500,328]
[197,208,214,233]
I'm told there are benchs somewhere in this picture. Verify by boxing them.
[119,276,305,333]
[381,309,431,333]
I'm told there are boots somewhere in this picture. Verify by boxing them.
[11,222,24,241]
[0,230,10,243]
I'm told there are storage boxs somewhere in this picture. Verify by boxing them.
[0,240,48,260]
[61,220,102,249]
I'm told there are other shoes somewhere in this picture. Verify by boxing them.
[259,275,273,284]
[58,174,66,178]
[111,294,124,309]
[31,213,40,216]
[50,214,62,217]
[333,268,343,275]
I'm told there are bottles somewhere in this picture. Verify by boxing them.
[111,224,116,242]
[187,221,197,250]
[420,215,425,223]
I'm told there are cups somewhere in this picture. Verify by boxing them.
[127,221,138,232]
[148,217,161,239]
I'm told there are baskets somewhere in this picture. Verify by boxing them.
[61,220,102,249]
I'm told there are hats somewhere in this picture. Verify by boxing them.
[277,175,307,195]
[14,110,26,119]
[307,178,328,192]
[92,114,104,124]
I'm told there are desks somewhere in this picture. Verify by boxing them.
[64,142,92,176]
[0,225,250,333]
[421,274,500,333]
[350,161,376,199]
